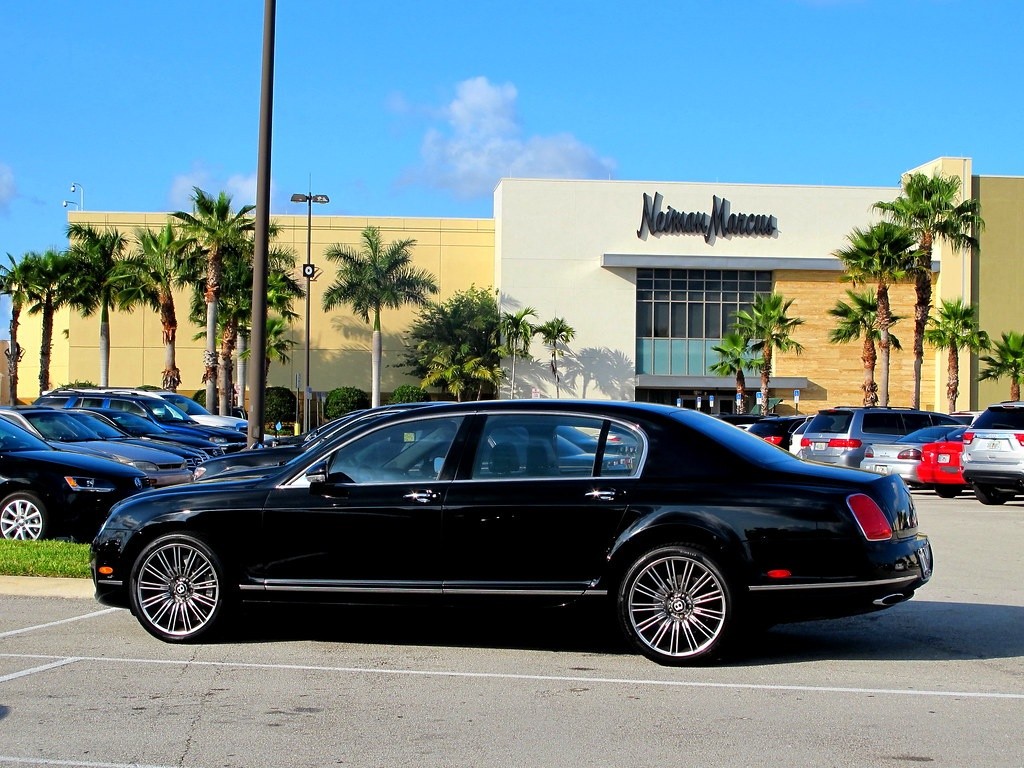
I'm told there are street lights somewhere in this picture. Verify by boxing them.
[291,191,330,438]
[62,200,79,210]
[71,182,84,212]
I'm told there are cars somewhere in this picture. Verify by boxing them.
[714,413,768,426]
[860,423,967,489]
[747,416,807,453]
[915,427,970,499]
[788,414,818,456]
[735,423,756,432]
[0,405,195,488]
[947,411,986,427]
[26,385,638,485]
[88,398,936,669]
[0,414,154,549]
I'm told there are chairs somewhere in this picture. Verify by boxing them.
[488,441,520,476]
[526,436,560,476]
[122,402,132,411]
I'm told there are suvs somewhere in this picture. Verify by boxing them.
[801,405,963,469]
[959,401,1024,505]
